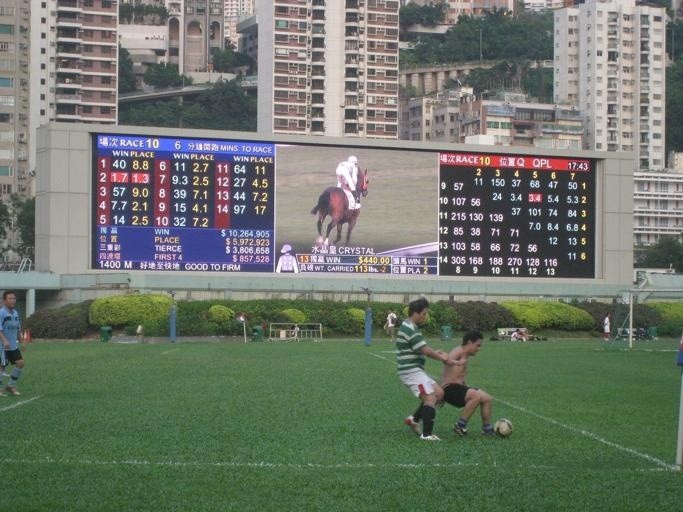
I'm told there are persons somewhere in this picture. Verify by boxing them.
[336,155,361,204]
[395,299,452,441]
[440,330,499,439]
[511,329,521,342]
[383,308,397,343]
[292,324,299,343]
[0,291,25,398]
[276,244,299,274]
[603,311,611,342]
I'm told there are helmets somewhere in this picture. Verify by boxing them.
[347,155,358,164]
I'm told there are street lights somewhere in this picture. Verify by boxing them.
[475,28,483,64]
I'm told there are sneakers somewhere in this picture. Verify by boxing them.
[349,203,361,210]
[452,423,469,438]
[403,415,421,436]
[0,385,20,398]
[420,432,441,442]
[481,430,500,437]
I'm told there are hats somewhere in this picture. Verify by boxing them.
[280,244,291,254]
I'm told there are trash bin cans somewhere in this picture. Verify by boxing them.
[648,327,657,337]
[100,327,112,342]
[252,326,262,342]
[441,326,451,341]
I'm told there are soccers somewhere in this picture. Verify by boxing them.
[494,419,513,438]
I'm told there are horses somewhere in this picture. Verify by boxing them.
[311,167,368,246]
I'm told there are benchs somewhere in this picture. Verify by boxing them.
[617,328,646,341]
[497,327,527,340]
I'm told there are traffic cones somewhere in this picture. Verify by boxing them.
[19,328,32,342]
